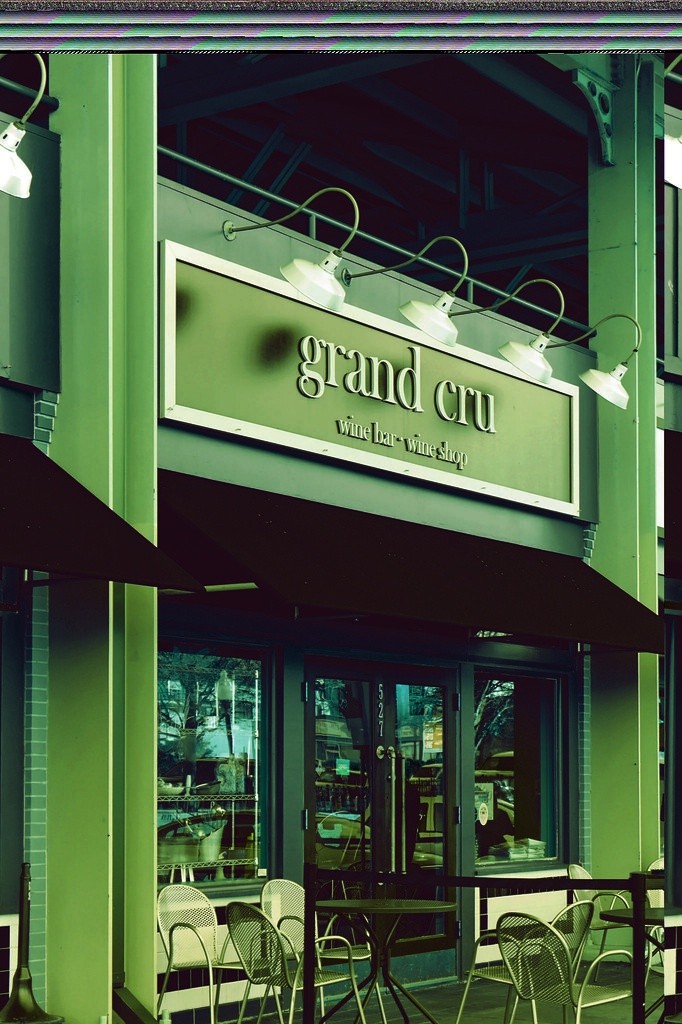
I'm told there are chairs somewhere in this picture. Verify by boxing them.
[158,884,285,1024]
[257,879,387,1024]
[225,901,367,1024]
[567,864,633,981]
[645,857,664,986]
[495,912,632,1024]
[456,900,595,1024]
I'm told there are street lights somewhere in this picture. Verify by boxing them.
[214,670,238,852]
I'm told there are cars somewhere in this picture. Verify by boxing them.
[359,798,516,844]
[156,810,442,884]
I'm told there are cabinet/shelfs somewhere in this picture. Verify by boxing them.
[157,664,258,883]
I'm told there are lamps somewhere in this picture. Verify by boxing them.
[222,187,360,311]
[544,314,642,409]
[448,279,565,384]
[341,236,468,347]
[0,54,47,199]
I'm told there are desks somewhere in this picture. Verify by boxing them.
[314,898,457,1024]
[599,907,682,1024]
